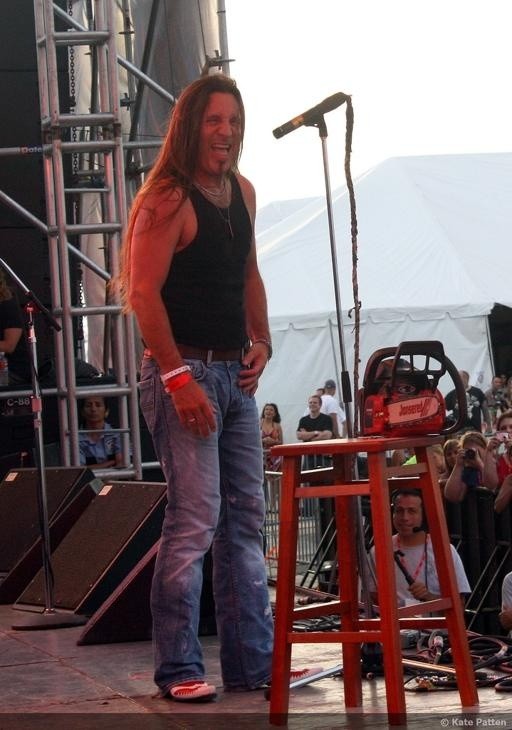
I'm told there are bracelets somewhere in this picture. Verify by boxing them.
[252,338,272,360]
[158,365,194,393]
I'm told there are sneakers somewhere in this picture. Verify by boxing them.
[169,681,218,700]
[256,668,322,684]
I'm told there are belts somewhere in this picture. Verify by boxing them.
[175,346,255,364]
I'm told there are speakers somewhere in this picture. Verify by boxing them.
[0,467,96,584]
[11,480,167,616]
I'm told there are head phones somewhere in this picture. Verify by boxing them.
[391,488,427,525]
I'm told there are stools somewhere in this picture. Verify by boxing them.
[269,432,482,728]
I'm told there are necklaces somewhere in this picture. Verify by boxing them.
[190,169,236,242]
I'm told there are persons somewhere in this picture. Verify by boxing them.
[0,272,37,468]
[121,75,323,698]
[73,391,129,470]
[258,403,284,471]
[295,370,512,636]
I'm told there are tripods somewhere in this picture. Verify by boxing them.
[264,139,488,701]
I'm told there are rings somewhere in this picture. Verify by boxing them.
[188,418,196,422]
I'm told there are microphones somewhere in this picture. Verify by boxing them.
[273,92,346,139]
[413,527,427,533]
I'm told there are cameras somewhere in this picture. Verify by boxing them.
[463,448,478,459]
[496,433,509,443]
